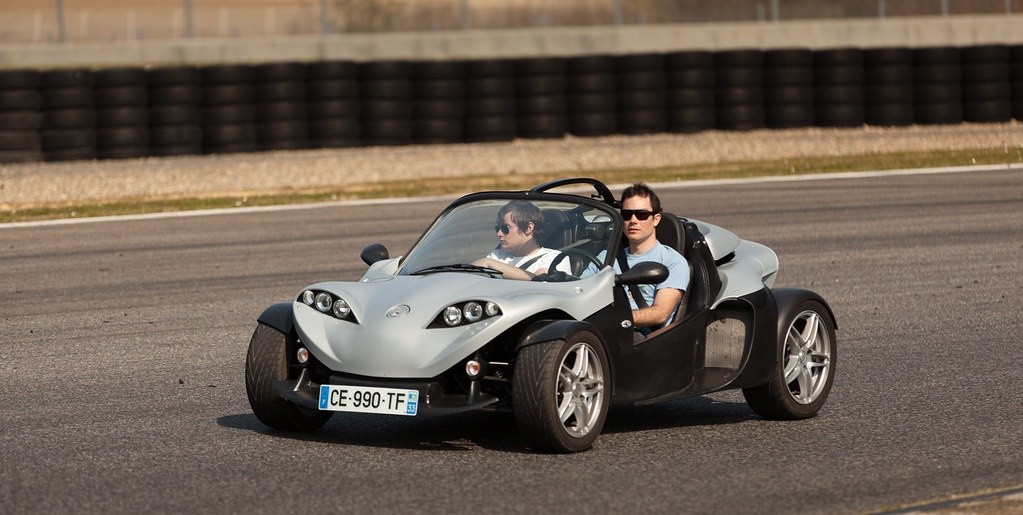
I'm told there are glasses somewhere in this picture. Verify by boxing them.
[495,224,516,234]
[621,209,654,221]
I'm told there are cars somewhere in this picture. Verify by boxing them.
[243,178,840,453]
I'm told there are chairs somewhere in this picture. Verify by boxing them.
[619,212,694,333]
[533,208,574,251]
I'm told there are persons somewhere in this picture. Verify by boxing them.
[580,184,690,342]
[465,200,572,283]
